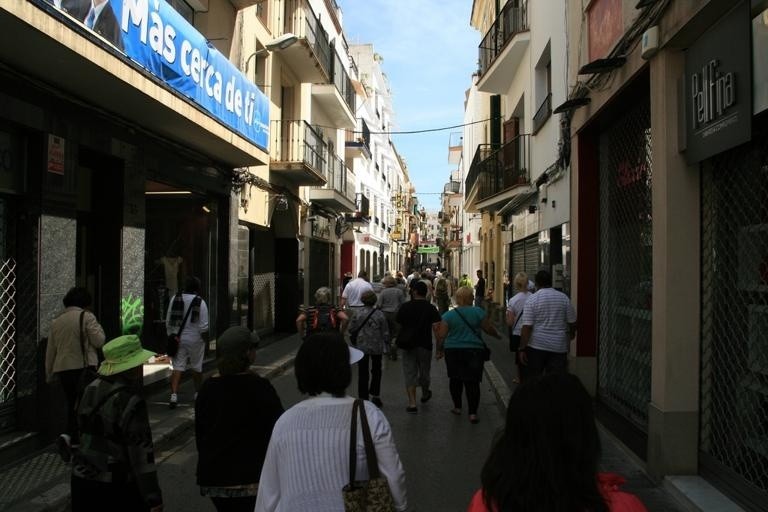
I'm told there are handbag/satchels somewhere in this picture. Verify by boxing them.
[340,474,397,512]
[348,330,358,346]
[75,369,104,389]
[155,324,178,357]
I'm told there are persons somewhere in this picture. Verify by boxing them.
[294,258,493,425]
[504,270,534,384]
[526,280,536,292]
[165,274,210,410]
[252,327,408,511]
[517,269,579,381]
[44,286,107,463]
[70,332,166,512]
[194,326,285,511]
[466,363,649,512]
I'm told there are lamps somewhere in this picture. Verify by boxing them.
[245,33,298,73]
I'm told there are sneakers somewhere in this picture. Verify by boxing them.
[406,405,418,414]
[450,408,462,416]
[168,393,179,407]
[469,414,477,421]
[420,390,432,403]
[371,397,383,409]
[57,435,71,463]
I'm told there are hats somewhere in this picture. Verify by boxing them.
[97,335,159,376]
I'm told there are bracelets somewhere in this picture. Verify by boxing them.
[518,347,526,352]
